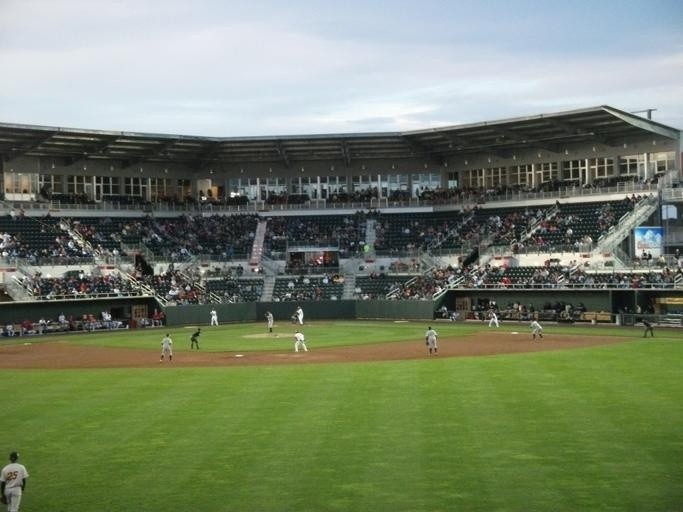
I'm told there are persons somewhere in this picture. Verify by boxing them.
[293,329,308,352]
[1,174,682,339]
[190,327,200,349]
[158,332,172,363]
[0,450,27,511]
[424,326,438,356]
